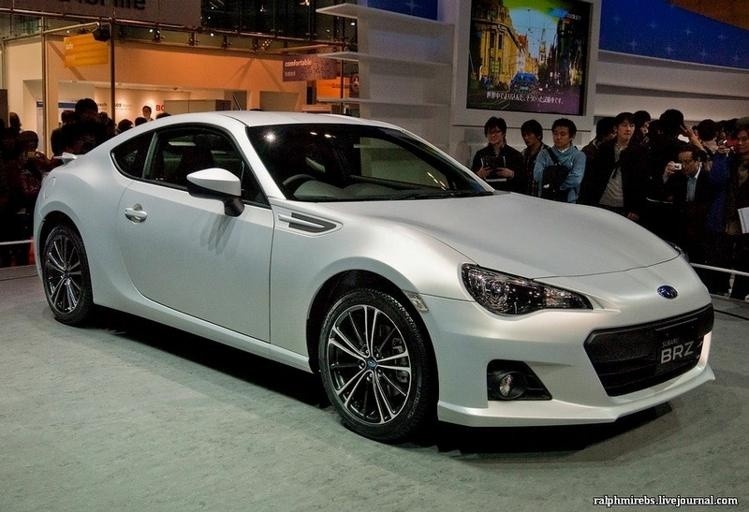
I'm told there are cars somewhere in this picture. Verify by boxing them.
[509,73,539,98]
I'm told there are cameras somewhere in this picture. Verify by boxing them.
[672,163,682,170]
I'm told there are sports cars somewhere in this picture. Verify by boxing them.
[27,108,718,444]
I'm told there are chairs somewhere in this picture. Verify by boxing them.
[170,143,217,187]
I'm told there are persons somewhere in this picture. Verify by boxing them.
[469,101,748,302]
[0,98,183,240]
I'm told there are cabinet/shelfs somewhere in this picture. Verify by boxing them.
[315,4,455,189]
